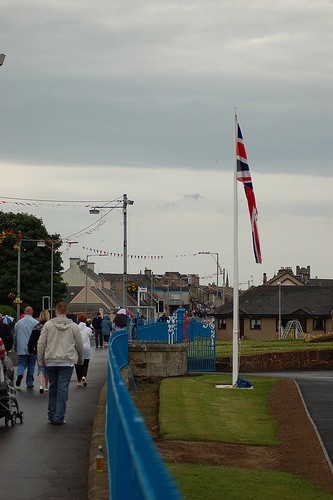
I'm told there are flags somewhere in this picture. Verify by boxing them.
[237,125,262,265]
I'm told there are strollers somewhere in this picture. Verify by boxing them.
[0,359,24,427]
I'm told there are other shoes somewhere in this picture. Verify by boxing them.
[47,420,66,425]
[81,377,87,387]
[76,381,81,386]
[15,375,23,387]
[26,385,33,389]
[39,386,48,393]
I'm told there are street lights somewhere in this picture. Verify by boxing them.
[199,252,218,307]
[84,254,109,318]
[51,242,78,319]
[276,282,281,339]
[89,205,128,311]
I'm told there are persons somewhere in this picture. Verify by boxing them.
[1,301,170,424]
